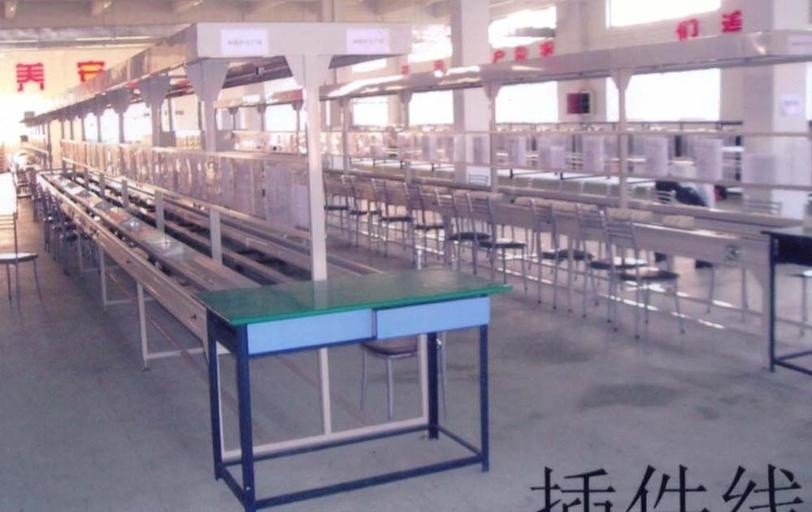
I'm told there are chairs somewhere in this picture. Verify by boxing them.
[1,149,795,419]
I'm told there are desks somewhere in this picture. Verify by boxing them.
[191,265,514,507]
[758,220,812,377]
